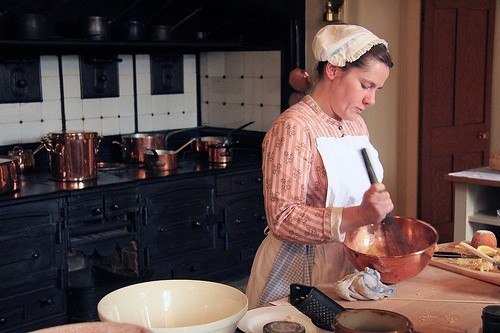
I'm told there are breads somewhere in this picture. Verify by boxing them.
[472,230,497,249]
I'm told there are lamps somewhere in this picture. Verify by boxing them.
[323,0,345,22]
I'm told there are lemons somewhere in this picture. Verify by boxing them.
[476,245,497,253]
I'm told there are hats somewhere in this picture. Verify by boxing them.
[311,24,389,67]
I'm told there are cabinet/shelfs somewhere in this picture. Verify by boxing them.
[0,197,69,333]
[444,165,500,242]
[66,185,139,322]
[141,176,227,283]
[216,169,269,284]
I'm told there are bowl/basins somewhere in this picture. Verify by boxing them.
[95,279,249,333]
[342,216,438,285]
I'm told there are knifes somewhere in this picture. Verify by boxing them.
[432,250,495,260]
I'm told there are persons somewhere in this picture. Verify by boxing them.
[245,25,394,310]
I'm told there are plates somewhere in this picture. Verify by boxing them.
[236,306,336,333]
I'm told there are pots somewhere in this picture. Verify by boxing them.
[40,130,104,182]
[10,0,205,42]
[111,133,163,164]
[143,146,179,172]
[193,119,262,167]
[0,144,36,195]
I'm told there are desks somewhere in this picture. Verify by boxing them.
[238,243,500,333]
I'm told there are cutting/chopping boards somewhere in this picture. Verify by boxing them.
[425,239,499,288]
